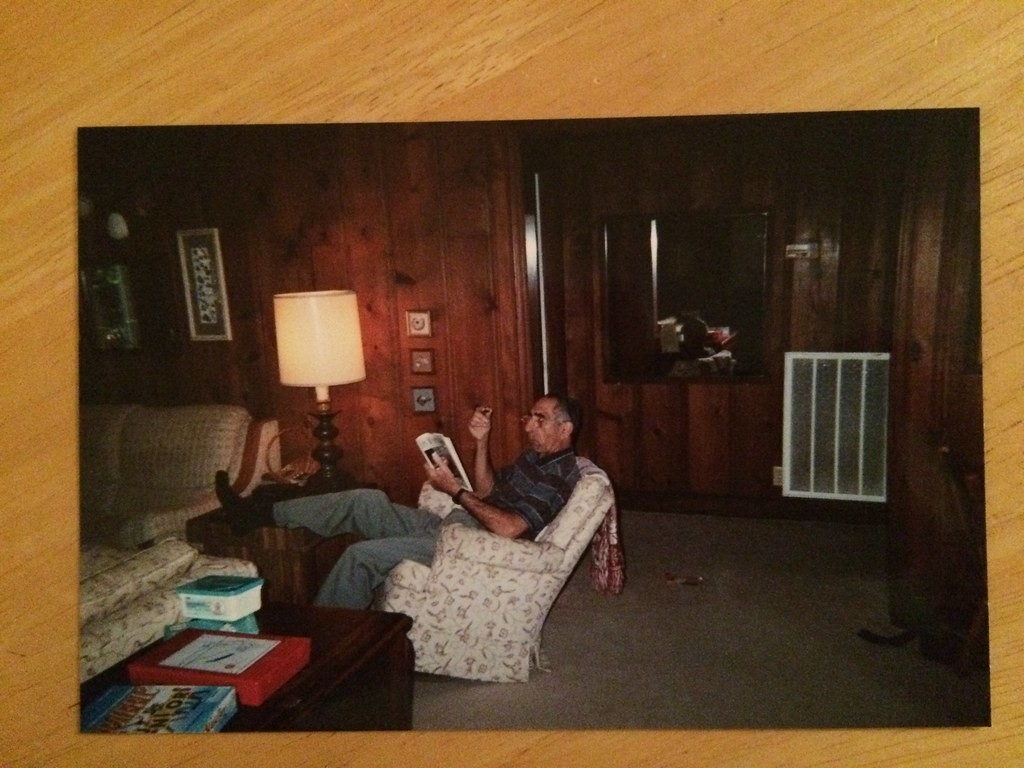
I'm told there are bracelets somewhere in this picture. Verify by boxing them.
[452,489,467,504]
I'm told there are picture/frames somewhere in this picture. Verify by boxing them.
[406,310,433,339]
[82,263,137,350]
[410,348,435,375]
[177,226,234,343]
[411,386,437,414]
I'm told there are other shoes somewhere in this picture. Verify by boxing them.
[215,471,253,537]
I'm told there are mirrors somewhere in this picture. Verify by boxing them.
[598,204,773,388]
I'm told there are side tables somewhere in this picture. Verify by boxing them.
[186,484,392,611]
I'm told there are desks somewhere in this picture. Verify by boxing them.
[711,327,741,359]
[80,602,415,734]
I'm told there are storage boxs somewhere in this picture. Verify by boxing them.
[125,627,313,708]
[176,573,263,621]
[81,684,239,734]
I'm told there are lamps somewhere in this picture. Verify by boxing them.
[272,288,367,489]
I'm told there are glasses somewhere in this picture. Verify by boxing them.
[522,412,569,428]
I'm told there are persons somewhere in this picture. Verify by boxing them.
[676,319,716,358]
[215,390,583,611]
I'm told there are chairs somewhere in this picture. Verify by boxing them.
[669,350,736,382]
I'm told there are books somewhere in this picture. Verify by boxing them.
[414,433,473,492]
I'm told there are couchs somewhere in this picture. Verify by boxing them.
[79,536,258,685]
[370,455,624,681]
[79,402,283,543]
